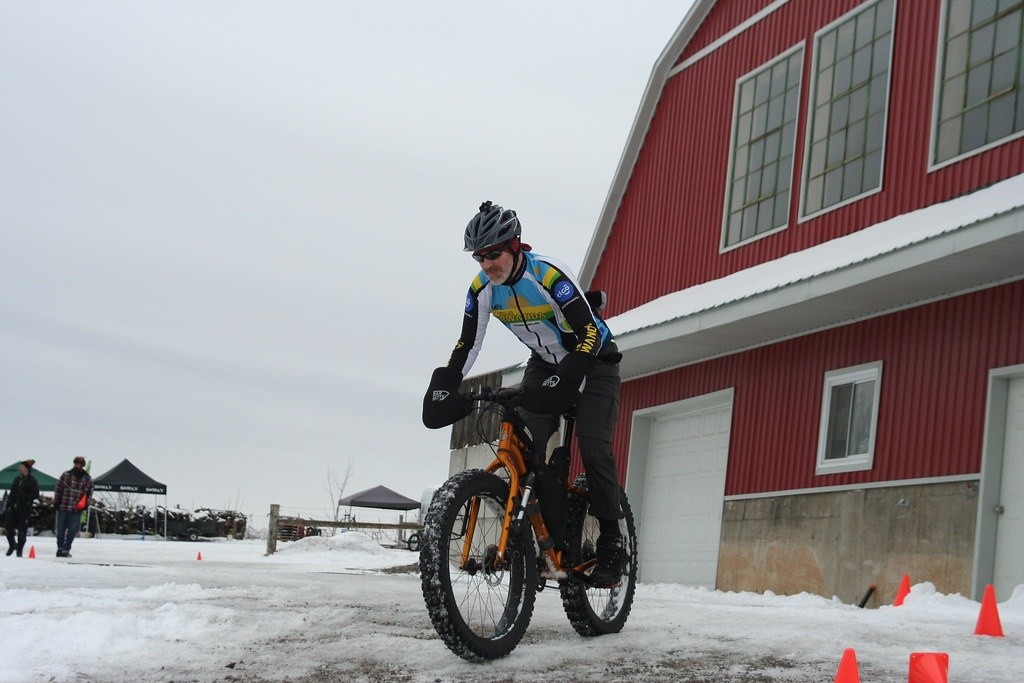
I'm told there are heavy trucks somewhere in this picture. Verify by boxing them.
[276,515,321,542]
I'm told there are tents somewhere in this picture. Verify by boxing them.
[0,461,58,491]
[89,458,167,541]
[336,485,422,549]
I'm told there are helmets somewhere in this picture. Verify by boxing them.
[461,202,522,252]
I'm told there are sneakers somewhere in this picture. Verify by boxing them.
[592,534,628,584]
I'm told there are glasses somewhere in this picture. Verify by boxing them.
[472,250,501,263]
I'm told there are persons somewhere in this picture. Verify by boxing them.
[424,200,629,589]
[52,456,94,558]
[3,460,39,558]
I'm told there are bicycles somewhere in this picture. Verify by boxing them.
[418,385,639,662]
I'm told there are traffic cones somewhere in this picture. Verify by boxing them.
[896,574,911,606]
[197,552,201,559]
[29,545,36,560]
[975,584,1004,637]
[834,647,860,683]
[909,654,950,683]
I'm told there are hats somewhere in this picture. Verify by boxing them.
[73,457,85,465]
[20,459,34,471]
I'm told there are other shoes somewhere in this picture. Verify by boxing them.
[56,548,69,557]
[6,543,24,557]
